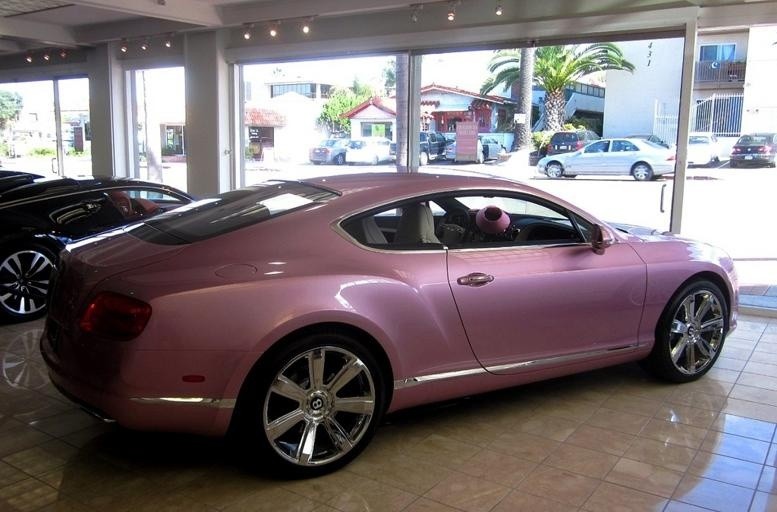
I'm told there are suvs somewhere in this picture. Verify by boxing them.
[546,131,601,155]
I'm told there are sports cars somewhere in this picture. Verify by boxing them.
[39,173,739,478]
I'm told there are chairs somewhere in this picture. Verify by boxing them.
[393,203,443,245]
[354,215,392,247]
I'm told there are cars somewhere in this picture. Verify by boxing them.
[625,135,668,151]
[0,177,193,321]
[537,139,687,181]
[527,144,548,167]
[674,131,719,167]
[0,169,46,192]
[345,138,393,166]
[728,131,777,168]
[308,138,347,166]
[64,379,726,512]
[1,317,83,424]
[444,136,505,164]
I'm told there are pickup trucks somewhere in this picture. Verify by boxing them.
[388,131,446,166]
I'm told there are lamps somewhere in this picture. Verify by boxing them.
[243,13,317,41]
[120,39,171,54]
[24,48,69,64]
[410,0,504,26]
